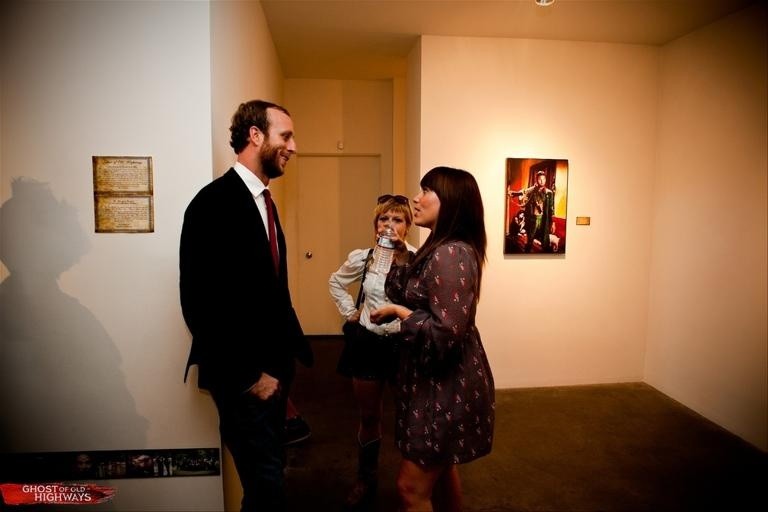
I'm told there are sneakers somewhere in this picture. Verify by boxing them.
[287,413,313,446]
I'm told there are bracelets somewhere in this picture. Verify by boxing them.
[392,242,407,254]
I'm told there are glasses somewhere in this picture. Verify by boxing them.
[377,193,410,205]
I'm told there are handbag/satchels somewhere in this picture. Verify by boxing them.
[334,317,359,378]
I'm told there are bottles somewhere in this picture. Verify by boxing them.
[370,220,398,274]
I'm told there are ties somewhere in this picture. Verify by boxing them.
[261,190,281,272]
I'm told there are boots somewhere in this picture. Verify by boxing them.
[348,430,383,507]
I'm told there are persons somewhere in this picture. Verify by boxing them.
[285,395,312,446]
[511,208,528,237]
[178,99,318,511]
[507,170,557,254]
[327,195,422,508]
[367,165,498,511]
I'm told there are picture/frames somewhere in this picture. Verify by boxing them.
[92,156,154,233]
